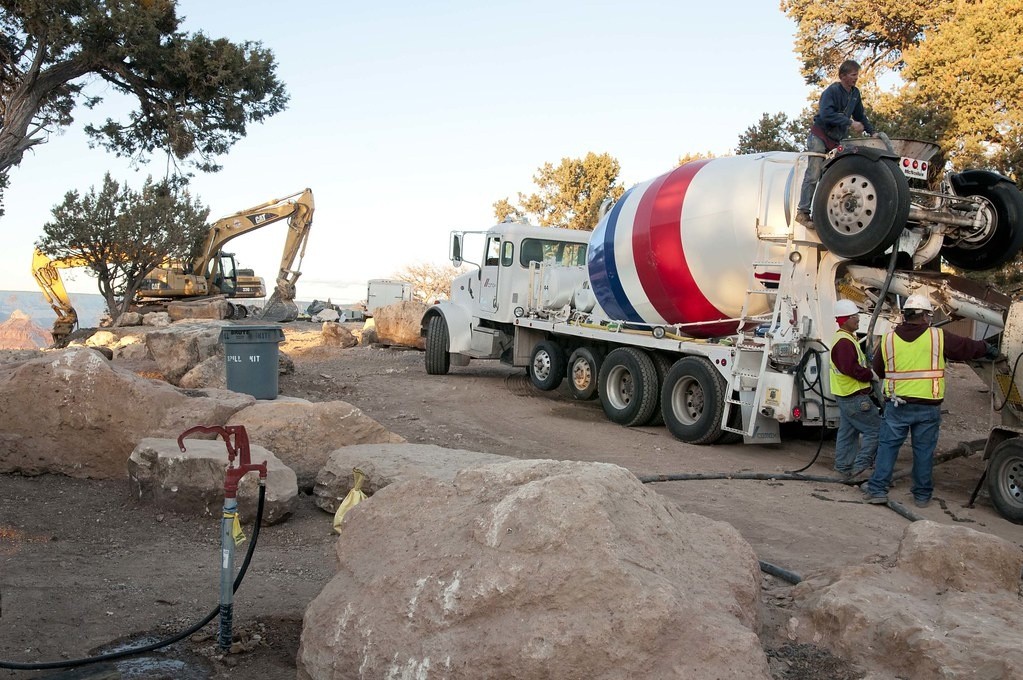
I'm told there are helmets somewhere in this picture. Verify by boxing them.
[833,299,860,316]
[903,294,934,316]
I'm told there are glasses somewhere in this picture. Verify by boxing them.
[849,314,860,322]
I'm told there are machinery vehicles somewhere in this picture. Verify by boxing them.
[130,188,316,323]
[30,239,267,347]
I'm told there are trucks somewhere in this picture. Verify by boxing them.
[360,278,415,322]
[964,299,1022,526]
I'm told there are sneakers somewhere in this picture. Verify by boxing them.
[852,466,875,482]
[795,214,814,229]
[833,468,852,478]
[860,492,888,503]
[913,496,930,508]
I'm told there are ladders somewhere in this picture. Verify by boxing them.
[720,230,795,438]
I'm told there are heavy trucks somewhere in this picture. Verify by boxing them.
[416,148,1023,447]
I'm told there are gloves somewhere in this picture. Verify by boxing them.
[871,369,880,383]
[891,394,906,407]
[986,342,999,360]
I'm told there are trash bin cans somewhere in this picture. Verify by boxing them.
[221,325,283,400]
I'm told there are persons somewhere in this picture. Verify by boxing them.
[795,60,878,229]
[860,294,1000,505]
[829,299,881,478]
